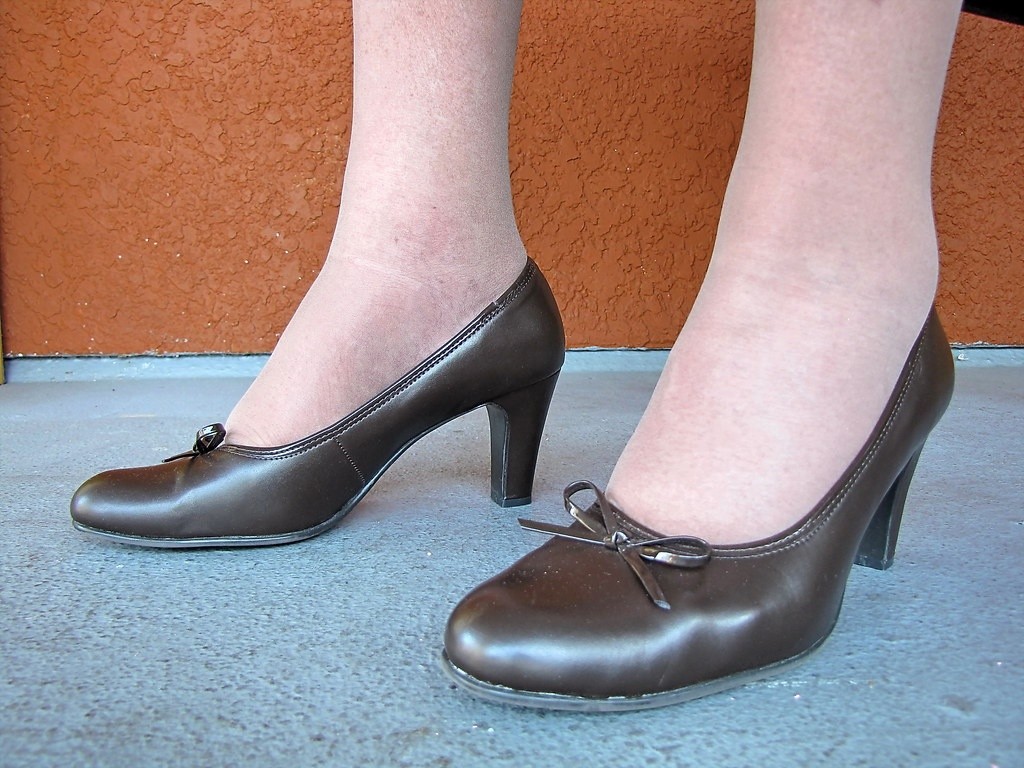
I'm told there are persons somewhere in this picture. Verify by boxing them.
[69,0,964,714]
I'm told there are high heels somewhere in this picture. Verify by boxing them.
[439,305,956,712]
[67,258,565,548]
